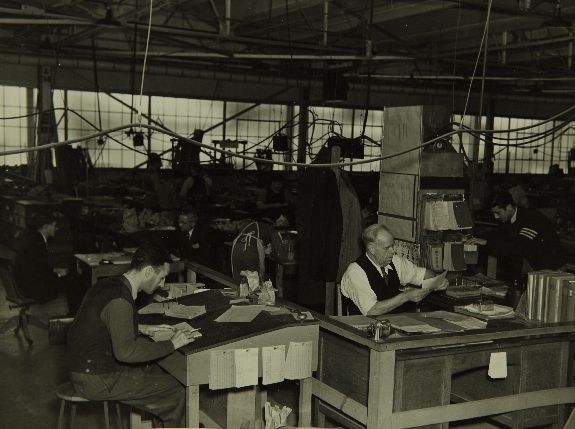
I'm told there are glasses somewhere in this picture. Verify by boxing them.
[177,220,195,224]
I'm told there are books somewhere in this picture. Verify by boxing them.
[528,270,575,323]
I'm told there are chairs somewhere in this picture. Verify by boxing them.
[324,282,351,317]
[0,267,50,344]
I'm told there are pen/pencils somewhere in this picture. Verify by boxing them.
[190,327,201,333]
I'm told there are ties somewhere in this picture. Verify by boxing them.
[186,231,190,240]
[381,266,389,285]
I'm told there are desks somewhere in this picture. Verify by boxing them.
[223,241,296,299]
[74,251,184,288]
[315,313,574,429]
[128,289,318,429]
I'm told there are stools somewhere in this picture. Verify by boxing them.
[56,383,123,429]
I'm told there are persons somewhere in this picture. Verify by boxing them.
[508,177,531,209]
[179,163,206,198]
[174,209,208,244]
[12,212,66,304]
[68,243,198,427]
[231,211,287,288]
[341,225,449,317]
[461,191,566,287]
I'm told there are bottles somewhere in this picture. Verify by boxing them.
[512,279,519,293]
